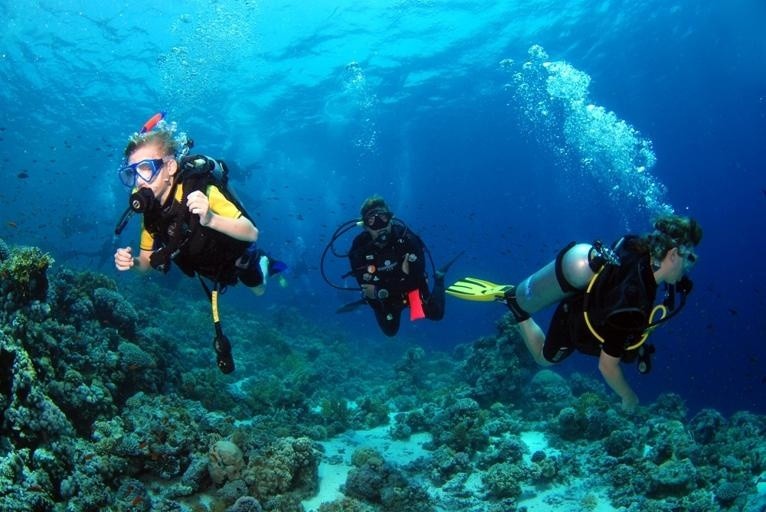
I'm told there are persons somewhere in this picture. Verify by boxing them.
[349,195,445,337]
[114,130,272,297]
[498,216,702,412]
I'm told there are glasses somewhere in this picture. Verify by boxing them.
[118,154,175,188]
[677,244,699,273]
[364,210,393,230]
